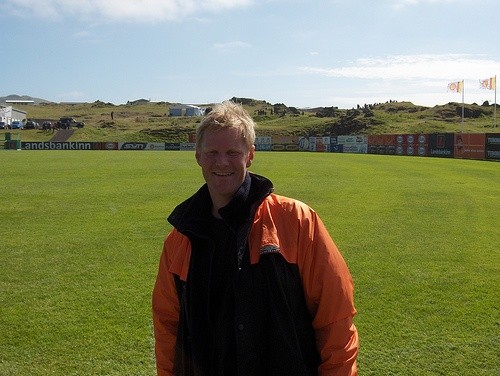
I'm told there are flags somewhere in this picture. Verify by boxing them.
[446,81,462,92]
[478,77,495,89]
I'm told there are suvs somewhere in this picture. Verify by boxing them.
[60,117,85,129]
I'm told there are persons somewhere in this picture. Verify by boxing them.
[152,98,360,376]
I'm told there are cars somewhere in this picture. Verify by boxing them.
[23,118,34,129]
[0,121,8,129]
[42,120,52,128]
[10,121,22,130]
